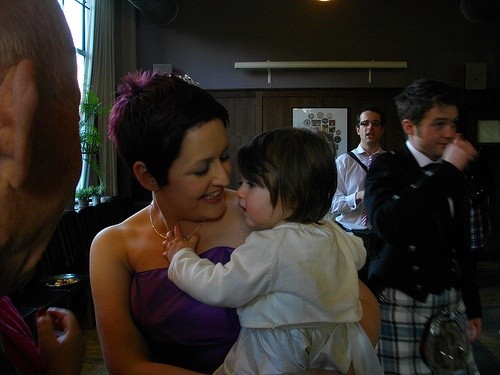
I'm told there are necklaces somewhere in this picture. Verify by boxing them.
[149,201,205,241]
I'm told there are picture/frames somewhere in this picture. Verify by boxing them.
[475,118,500,145]
[465,62,487,91]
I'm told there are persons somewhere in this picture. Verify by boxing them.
[89,71,381,375]
[453,129,486,273]
[331,106,394,284]
[363,79,482,375]
[162,127,384,375]
[0,0,86,375]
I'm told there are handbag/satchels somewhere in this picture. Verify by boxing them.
[420,304,469,370]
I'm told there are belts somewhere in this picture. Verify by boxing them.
[337,223,376,235]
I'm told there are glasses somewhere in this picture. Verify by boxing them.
[357,120,381,127]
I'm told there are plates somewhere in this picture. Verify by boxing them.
[44,274,79,287]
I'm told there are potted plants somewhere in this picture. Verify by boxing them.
[75,80,112,213]
